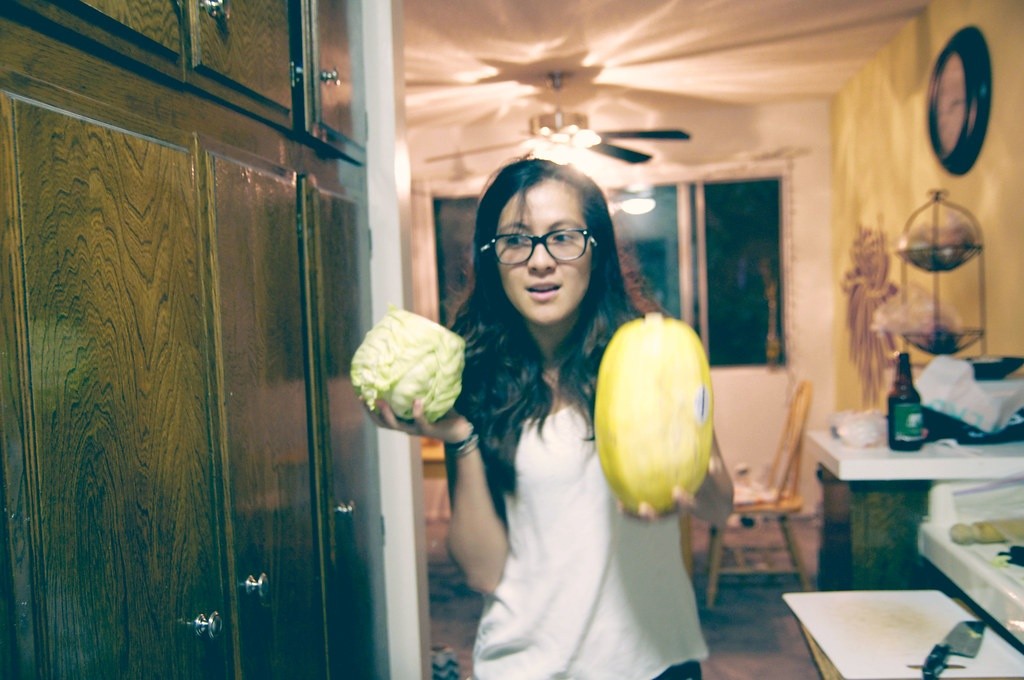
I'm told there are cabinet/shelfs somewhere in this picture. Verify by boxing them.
[0,0,396,680]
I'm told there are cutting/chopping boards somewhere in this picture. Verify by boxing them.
[782,590,1024,680]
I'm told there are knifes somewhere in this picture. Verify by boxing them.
[922,621,987,680]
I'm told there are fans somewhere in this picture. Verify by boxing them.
[423,71,691,185]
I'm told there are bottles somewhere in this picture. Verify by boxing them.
[888,353,924,451]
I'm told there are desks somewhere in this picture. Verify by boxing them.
[807,431,1024,596]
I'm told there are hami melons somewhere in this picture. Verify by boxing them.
[593,312,714,515]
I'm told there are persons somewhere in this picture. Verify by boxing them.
[360,159,736,679]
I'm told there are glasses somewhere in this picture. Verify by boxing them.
[481,228,598,266]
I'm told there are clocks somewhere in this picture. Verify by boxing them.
[928,25,993,176]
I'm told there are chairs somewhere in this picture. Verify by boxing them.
[705,381,815,608]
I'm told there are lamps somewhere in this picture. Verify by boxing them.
[528,108,595,169]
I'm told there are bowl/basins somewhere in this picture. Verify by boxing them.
[965,356,1024,381]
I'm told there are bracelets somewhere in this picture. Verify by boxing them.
[446,423,479,456]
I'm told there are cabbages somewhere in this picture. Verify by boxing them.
[349,306,466,424]
